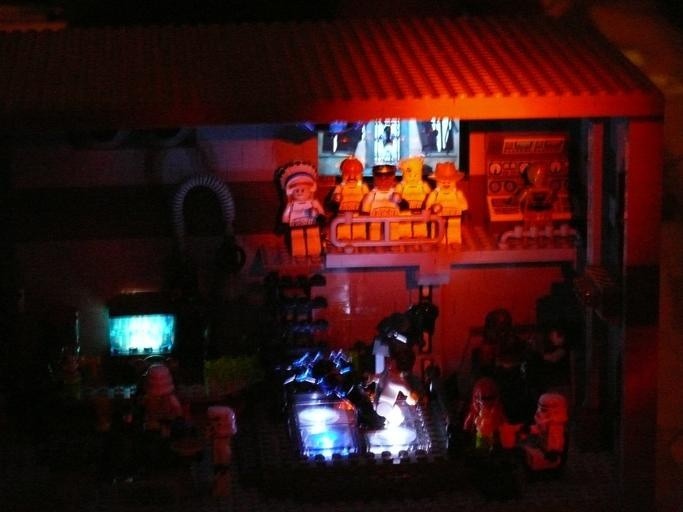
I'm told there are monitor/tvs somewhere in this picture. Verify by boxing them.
[316,117,466,179]
[105,310,181,362]
